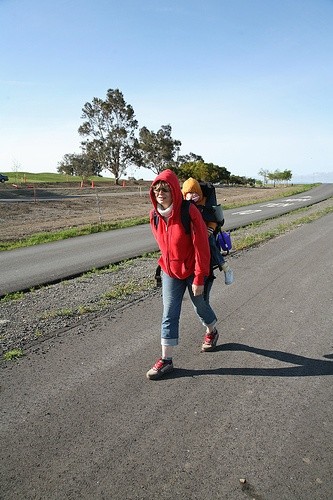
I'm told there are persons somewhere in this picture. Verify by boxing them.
[146,169,219,380]
[181,177,235,286]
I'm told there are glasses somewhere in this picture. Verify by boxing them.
[152,186,170,193]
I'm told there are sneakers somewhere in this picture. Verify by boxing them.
[225,269,233,285]
[147,356,173,380]
[202,327,219,352]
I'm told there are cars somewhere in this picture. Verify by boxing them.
[0,174,8,184]
